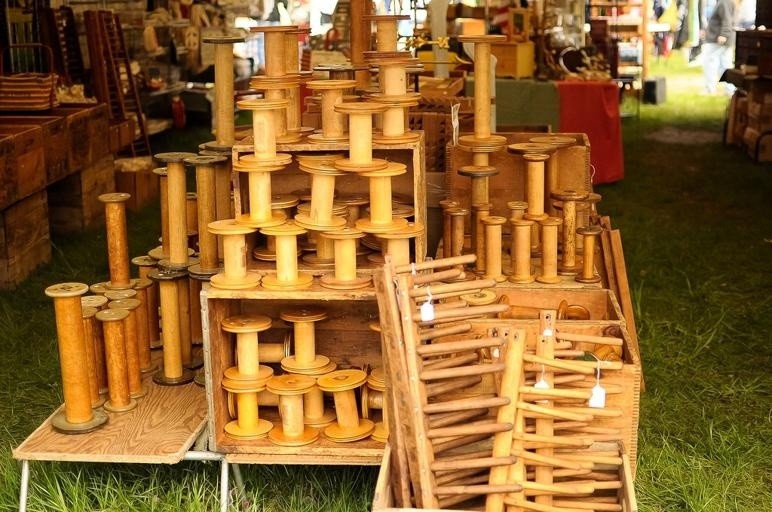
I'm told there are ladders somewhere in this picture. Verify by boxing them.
[49,5,152,158]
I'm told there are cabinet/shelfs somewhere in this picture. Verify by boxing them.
[723,29,772,164]
[0,0,654,512]
